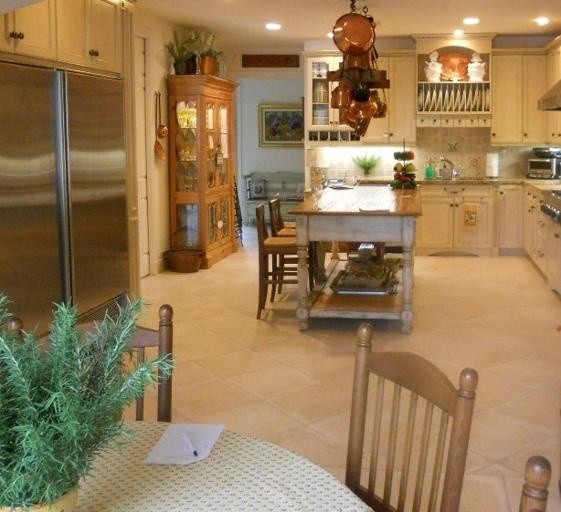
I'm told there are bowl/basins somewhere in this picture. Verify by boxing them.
[532,147,561,159]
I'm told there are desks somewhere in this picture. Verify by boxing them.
[77,422,380,512]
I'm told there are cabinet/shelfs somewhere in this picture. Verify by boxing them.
[491,48,545,147]
[545,35,561,97]
[2,0,55,67]
[359,53,418,145]
[414,185,499,259]
[303,49,359,145]
[418,81,491,129]
[500,183,525,256]
[549,215,561,298]
[165,74,242,270]
[53,0,123,79]
[526,184,550,278]
[546,110,561,145]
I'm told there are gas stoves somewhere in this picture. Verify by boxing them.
[539,189,561,223]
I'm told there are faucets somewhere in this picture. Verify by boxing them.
[440,156,455,178]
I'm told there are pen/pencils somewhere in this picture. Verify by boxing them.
[182,431,197,457]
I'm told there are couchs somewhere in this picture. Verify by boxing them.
[240,173,306,226]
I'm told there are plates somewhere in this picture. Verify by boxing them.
[174,101,228,189]
[416,84,491,112]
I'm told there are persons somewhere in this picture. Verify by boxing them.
[268,114,282,140]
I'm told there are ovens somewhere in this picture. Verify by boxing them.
[547,219,561,295]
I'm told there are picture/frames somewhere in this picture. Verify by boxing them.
[258,104,305,147]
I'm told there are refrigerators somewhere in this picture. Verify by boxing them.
[0,50,130,357]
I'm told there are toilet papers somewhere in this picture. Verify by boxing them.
[486,153,499,178]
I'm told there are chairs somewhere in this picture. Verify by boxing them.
[5,304,176,423]
[255,205,314,318]
[271,198,322,294]
[343,323,479,511]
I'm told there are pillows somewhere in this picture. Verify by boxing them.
[247,179,266,198]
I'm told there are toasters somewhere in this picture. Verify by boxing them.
[527,159,560,178]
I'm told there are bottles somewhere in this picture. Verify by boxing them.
[424,162,449,179]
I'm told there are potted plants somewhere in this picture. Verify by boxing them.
[354,154,384,177]
[167,30,198,75]
[0,292,176,512]
[198,32,227,78]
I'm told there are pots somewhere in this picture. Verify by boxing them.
[323,0,390,134]
